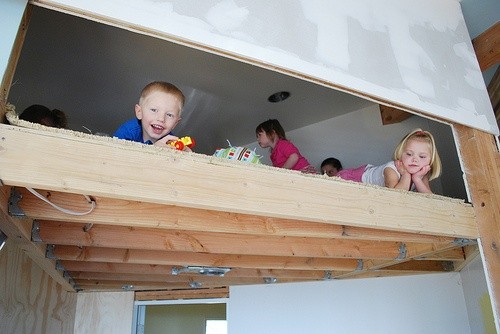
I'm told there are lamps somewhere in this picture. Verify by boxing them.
[268,91,290,103]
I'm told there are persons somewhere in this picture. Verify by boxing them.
[320,157,343,177]
[18,104,66,128]
[334,127,442,194]
[256,118,320,174]
[113,81,192,152]
